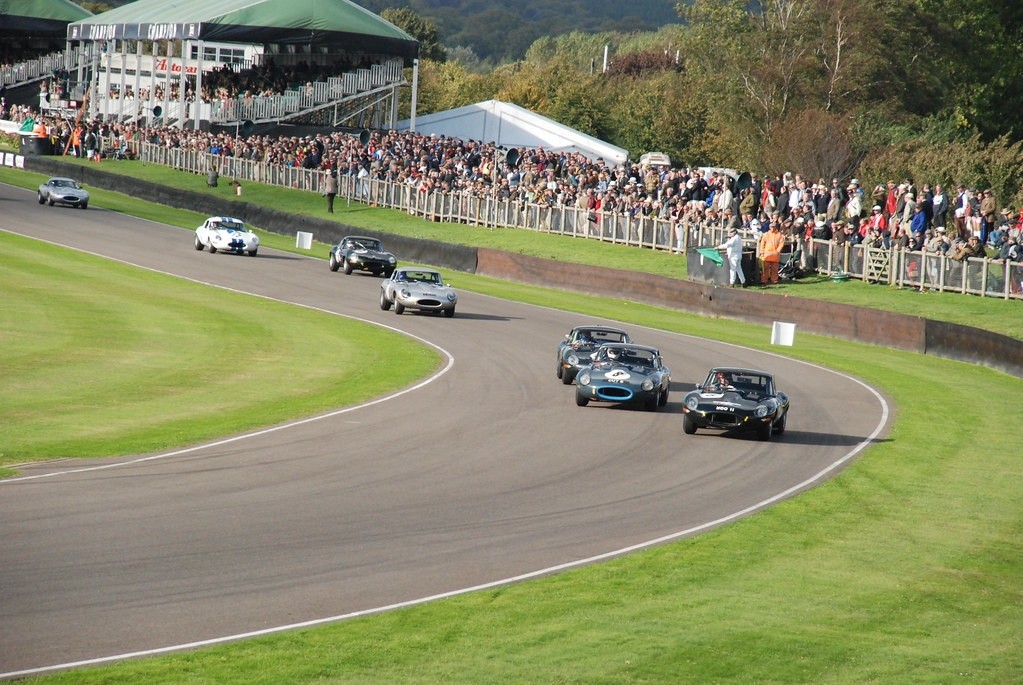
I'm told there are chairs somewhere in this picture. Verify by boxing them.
[715,378,734,387]
[744,383,762,400]
[633,357,651,368]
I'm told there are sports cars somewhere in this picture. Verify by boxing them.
[575,343,670,408]
[381,267,457,318]
[682,366,789,439]
[556,324,632,386]
[327,235,397,278]
[38,177,89,209]
[193,216,259,257]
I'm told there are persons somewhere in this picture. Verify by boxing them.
[760,222,785,287]
[36,115,146,163]
[774,170,1023,301]
[212,223,219,228]
[147,123,265,181]
[715,372,735,390]
[324,169,338,213]
[565,150,780,254]
[603,348,623,363]
[266,128,402,208]
[579,331,599,343]
[397,271,408,282]
[347,239,359,250]
[206,166,219,188]
[715,228,747,288]
[0,42,107,90]
[0,68,70,127]
[398,131,569,238]
[109,56,381,122]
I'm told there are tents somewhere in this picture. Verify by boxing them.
[66,1,421,133]
